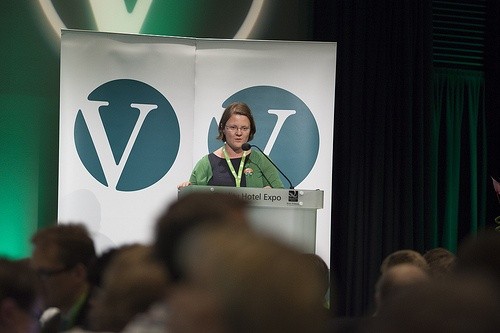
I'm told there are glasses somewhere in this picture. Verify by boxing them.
[30,264,75,280]
[221,123,251,134]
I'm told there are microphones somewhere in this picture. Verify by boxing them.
[241,143,294,189]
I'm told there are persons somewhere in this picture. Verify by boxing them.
[1,195,500,333]
[177,103,284,189]
